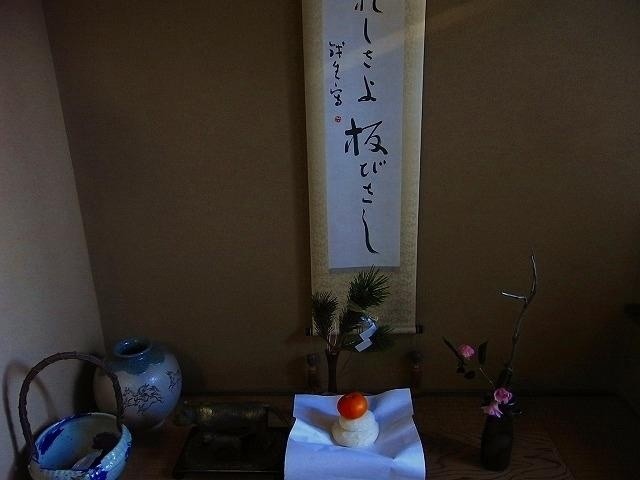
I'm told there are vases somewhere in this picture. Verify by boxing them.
[481,396,518,471]
[92,336,184,431]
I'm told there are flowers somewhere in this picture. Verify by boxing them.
[444,249,538,418]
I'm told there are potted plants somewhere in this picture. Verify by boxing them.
[309,264,400,394]
